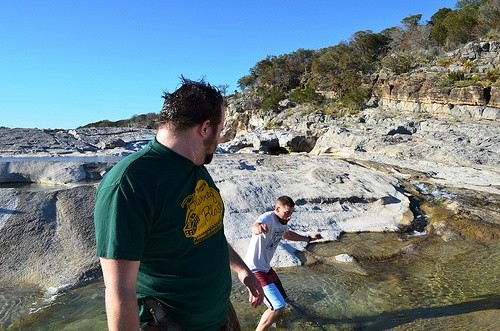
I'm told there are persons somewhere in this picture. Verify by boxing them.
[244,196,324,331]
[93,72,264,331]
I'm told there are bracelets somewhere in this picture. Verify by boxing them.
[307,235,311,243]
